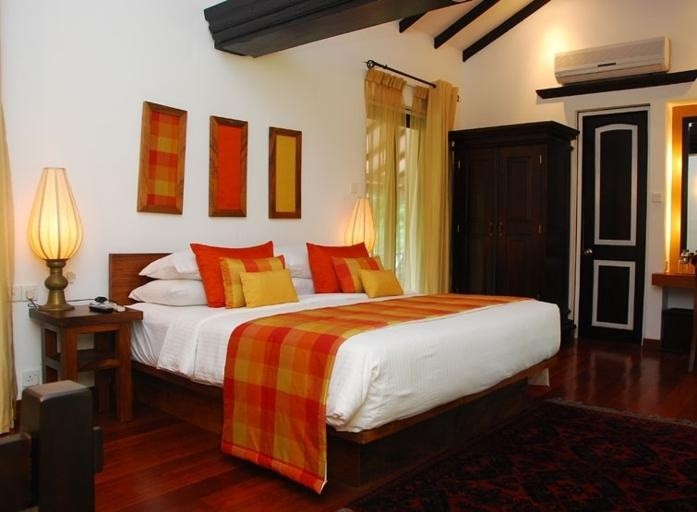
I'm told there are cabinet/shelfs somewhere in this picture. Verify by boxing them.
[448,120,580,351]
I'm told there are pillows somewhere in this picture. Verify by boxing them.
[128,280,208,307]
[306,241,370,294]
[331,254,385,294]
[138,245,203,281]
[239,269,296,308]
[218,254,285,309]
[189,242,274,307]
[358,268,401,299]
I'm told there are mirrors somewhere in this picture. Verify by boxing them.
[663,99,697,265]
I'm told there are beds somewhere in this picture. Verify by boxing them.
[109,249,562,486]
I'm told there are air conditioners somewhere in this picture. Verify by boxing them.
[553,36,670,86]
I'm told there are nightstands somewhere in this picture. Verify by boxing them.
[29,303,143,423]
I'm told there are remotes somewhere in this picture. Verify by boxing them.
[88,303,115,314]
[102,300,127,312]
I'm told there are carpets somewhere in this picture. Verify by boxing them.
[331,396,697,511]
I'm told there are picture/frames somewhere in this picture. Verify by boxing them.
[209,115,248,218]
[268,125,302,219]
[137,100,188,214]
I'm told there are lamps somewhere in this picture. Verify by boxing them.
[343,198,376,254]
[26,167,83,313]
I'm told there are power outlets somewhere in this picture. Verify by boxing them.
[21,286,39,303]
